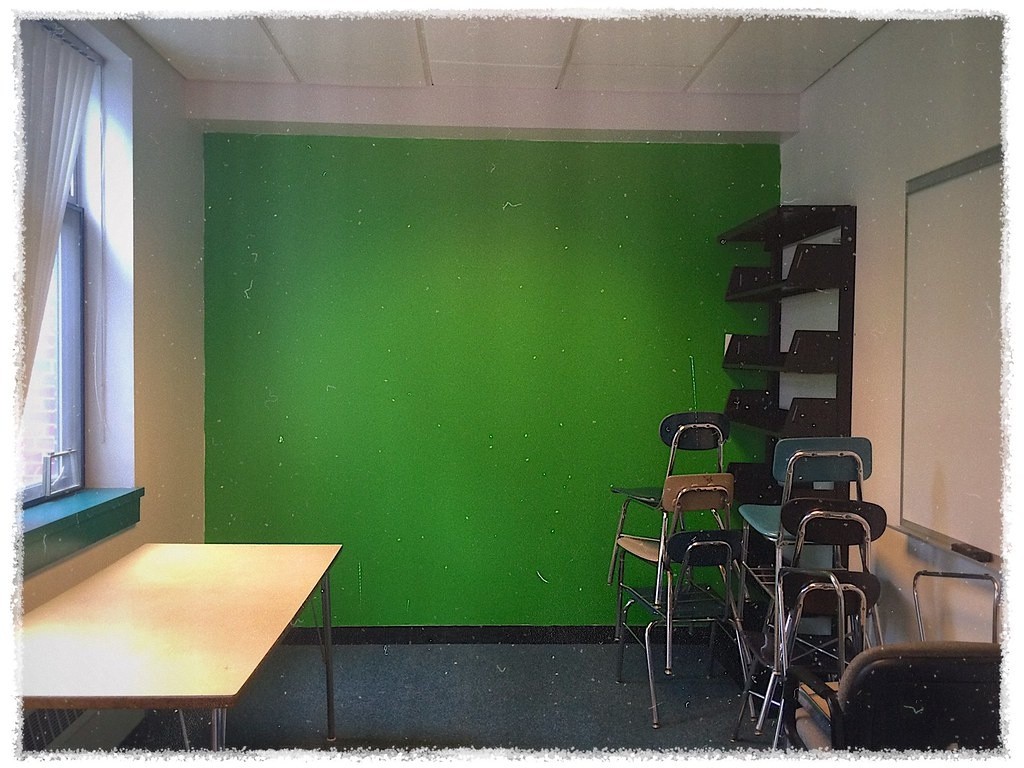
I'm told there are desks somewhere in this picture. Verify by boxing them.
[16,541,343,752]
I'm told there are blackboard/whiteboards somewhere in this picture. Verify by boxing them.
[901,146,1002,576]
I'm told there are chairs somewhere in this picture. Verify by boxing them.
[606,411,1001,752]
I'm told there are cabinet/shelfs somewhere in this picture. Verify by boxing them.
[715,203,859,722]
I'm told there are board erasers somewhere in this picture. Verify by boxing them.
[951,542,993,563]
